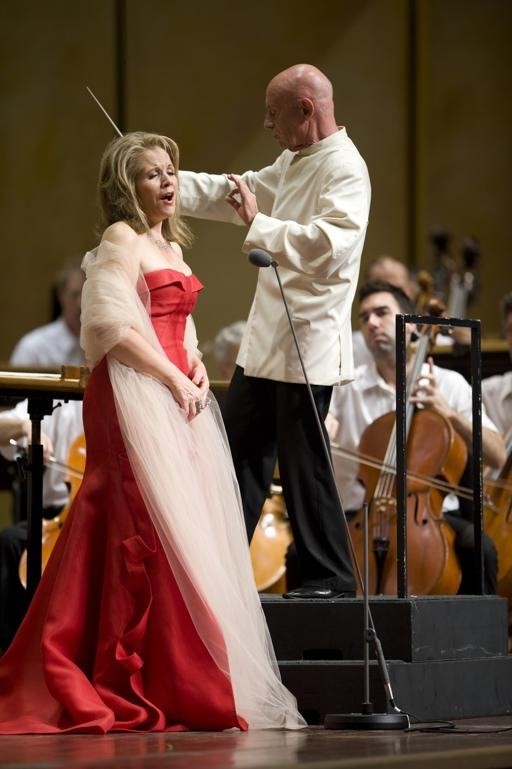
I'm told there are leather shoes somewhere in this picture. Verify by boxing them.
[282,586,356,599]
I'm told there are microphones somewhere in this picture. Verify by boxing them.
[248,247,277,269]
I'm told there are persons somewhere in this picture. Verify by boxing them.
[171,64,374,598]
[216,320,247,385]
[1,132,308,736]
[0,256,87,524]
[319,256,512,595]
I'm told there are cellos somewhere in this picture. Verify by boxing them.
[345,271,462,596]
[482,429,512,595]
[18,428,87,590]
